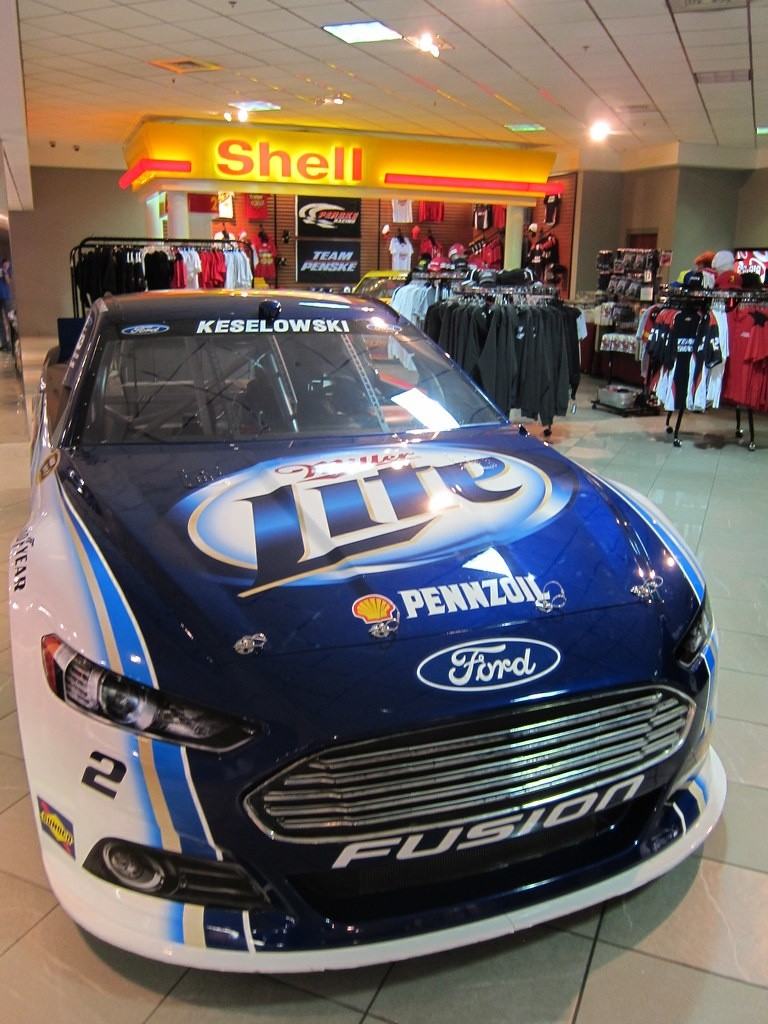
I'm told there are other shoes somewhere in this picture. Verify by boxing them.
[0,345,9,352]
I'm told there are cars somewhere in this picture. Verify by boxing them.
[8,288,728,975]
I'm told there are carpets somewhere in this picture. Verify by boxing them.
[657,289,768,313]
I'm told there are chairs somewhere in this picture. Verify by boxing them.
[236,348,326,437]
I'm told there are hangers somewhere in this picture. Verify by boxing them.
[77,235,244,262]
[405,272,560,312]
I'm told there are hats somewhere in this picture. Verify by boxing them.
[412,243,533,287]
[665,249,763,291]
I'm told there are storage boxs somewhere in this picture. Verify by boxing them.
[596,383,644,409]
